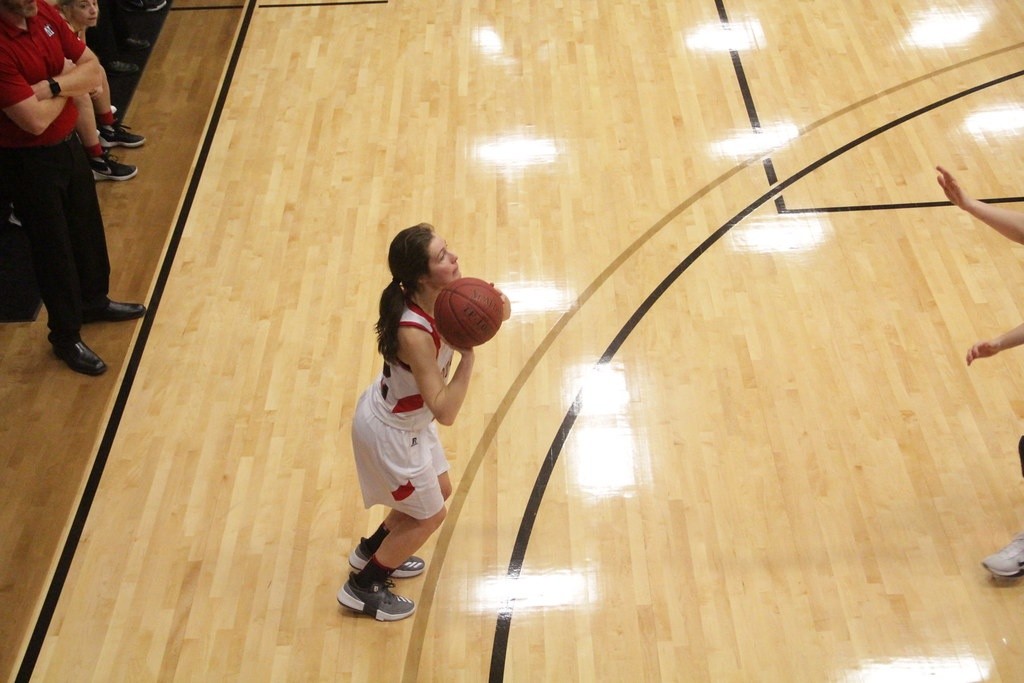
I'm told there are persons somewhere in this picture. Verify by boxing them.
[936,165,1024,578]
[0,0,147,374]
[338,222,511,621]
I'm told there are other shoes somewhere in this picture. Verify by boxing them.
[118,37,150,51]
[144,0,167,12]
[8,203,22,226]
[104,61,139,75]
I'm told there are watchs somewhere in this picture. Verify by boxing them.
[48,78,61,99]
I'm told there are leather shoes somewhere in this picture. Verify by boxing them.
[53,341,106,374]
[82,300,146,324]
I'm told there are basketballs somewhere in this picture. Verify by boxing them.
[434,277,504,350]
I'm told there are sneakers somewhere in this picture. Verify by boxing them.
[89,153,138,182]
[982,533,1024,577]
[98,121,146,147]
[337,571,416,621]
[349,537,426,578]
